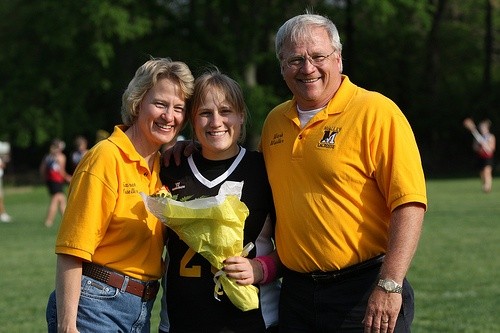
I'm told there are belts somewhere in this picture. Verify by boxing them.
[82,264,160,302]
[281,254,384,284]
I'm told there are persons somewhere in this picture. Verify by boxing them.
[158,64,285,331]
[73,137,90,165]
[473,120,496,192]
[0,140,14,222]
[46,57,195,332]
[159,6,427,332]
[41,139,75,227]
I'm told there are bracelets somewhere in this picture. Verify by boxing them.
[253,255,277,283]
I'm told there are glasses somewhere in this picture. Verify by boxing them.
[281,49,338,67]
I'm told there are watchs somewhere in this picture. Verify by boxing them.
[378,278,403,293]
